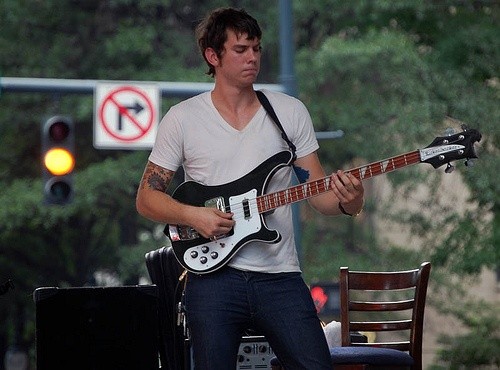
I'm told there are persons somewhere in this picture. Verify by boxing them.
[137,6,365,370]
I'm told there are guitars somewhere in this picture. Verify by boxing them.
[165,122,484,278]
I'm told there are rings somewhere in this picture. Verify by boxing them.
[212,235,216,239]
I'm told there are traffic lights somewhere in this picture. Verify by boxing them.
[38,113,79,206]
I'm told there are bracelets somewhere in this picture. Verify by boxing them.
[338,201,363,217]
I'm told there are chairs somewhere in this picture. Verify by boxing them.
[271,261,432,370]
